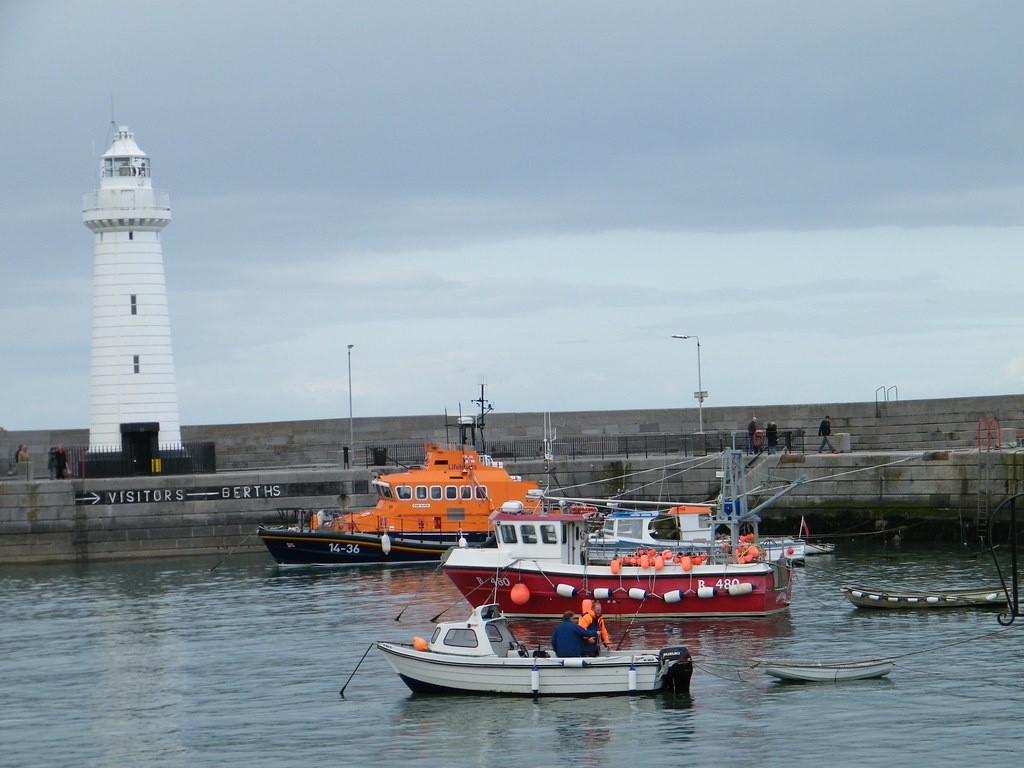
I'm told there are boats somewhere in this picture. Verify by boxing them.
[839,585,1012,611]
[805,543,835,555]
[255,404,561,567]
[375,566,693,696]
[440,432,794,620]
[751,655,894,682]
[528,502,806,568]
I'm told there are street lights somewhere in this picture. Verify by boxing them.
[347,345,353,467]
[671,335,702,433]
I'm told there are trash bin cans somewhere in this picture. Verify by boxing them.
[692,432,707,457]
[372,446,387,466]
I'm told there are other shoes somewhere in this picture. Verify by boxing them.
[759,449,763,452]
[769,453,776,455]
[818,451,822,453]
[749,452,753,455]
[831,449,837,453]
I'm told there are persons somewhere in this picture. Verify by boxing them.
[818,416,837,455]
[766,422,777,456]
[15,445,29,461]
[748,417,759,454]
[551,612,602,658]
[578,603,611,657]
[48,447,66,479]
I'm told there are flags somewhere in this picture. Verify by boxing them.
[802,518,809,538]
